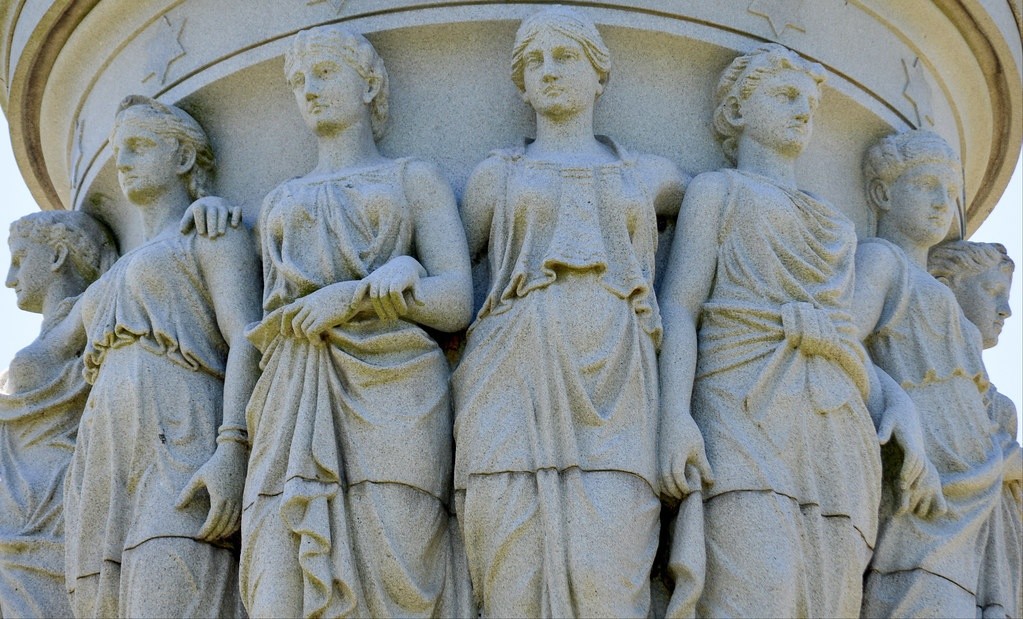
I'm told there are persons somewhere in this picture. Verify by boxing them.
[1,11,1023,619]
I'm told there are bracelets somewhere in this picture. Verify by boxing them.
[216,434,251,448]
[219,422,246,435]
